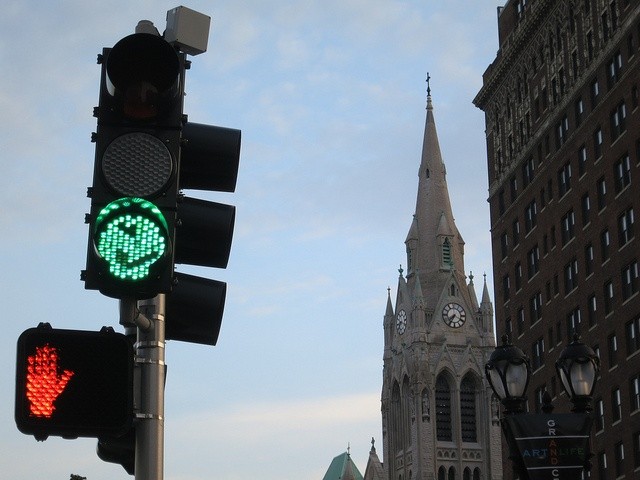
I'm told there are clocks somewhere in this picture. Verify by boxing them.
[441,302,466,328]
[395,308,407,335]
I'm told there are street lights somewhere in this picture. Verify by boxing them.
[485,334,598,480]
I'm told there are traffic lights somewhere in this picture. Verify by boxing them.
[15,323,135,463]
[165,122,241,346]
[80,33,188,299]
